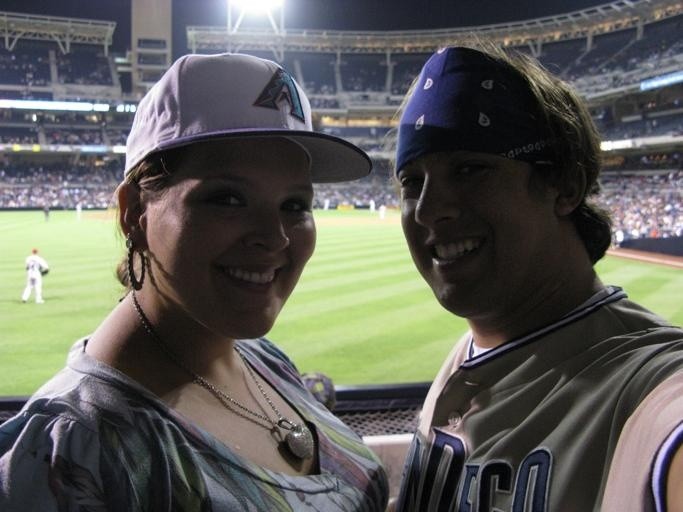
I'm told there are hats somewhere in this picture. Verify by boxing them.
[123,52,374,185]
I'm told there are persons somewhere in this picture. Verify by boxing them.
[0,30,682,249]
[0,50,390,510]
[390,40,682,510]
[18,247,49,307]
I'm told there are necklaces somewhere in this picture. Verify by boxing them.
[126,288,314,467]
